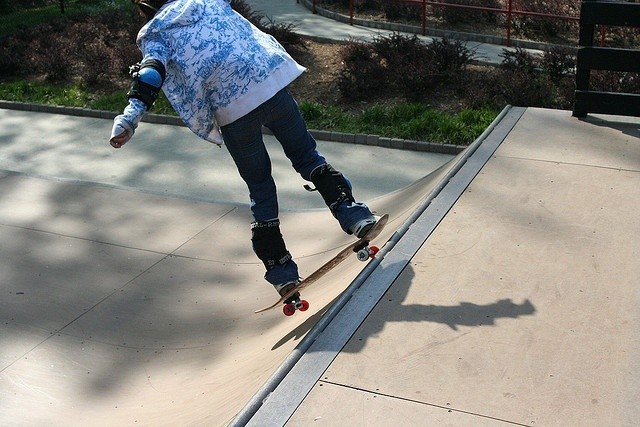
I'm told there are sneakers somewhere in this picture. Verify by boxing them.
[271,276,304,296]
[350,215,382,238]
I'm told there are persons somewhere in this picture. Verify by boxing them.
[108,0,383,297]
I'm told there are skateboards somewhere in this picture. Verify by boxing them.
[254,212,389,316]
